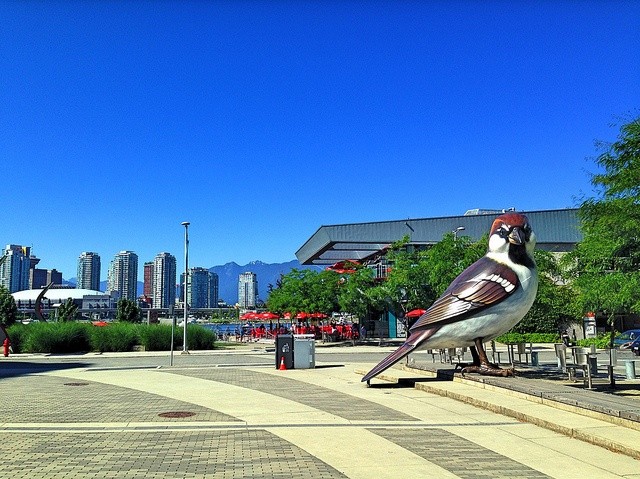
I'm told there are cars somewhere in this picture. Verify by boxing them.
[605,329,640,355]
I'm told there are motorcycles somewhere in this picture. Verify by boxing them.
[561,330,570,347]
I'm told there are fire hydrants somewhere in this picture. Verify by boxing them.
[3,339,10,356]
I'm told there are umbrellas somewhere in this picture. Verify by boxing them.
[239,309,326,339]
[406,309,426,319]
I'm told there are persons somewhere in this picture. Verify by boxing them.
[221,318,365,341]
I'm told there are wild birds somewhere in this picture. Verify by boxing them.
[360,211,539,385]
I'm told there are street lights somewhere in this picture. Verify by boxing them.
[181,221,190,355]
